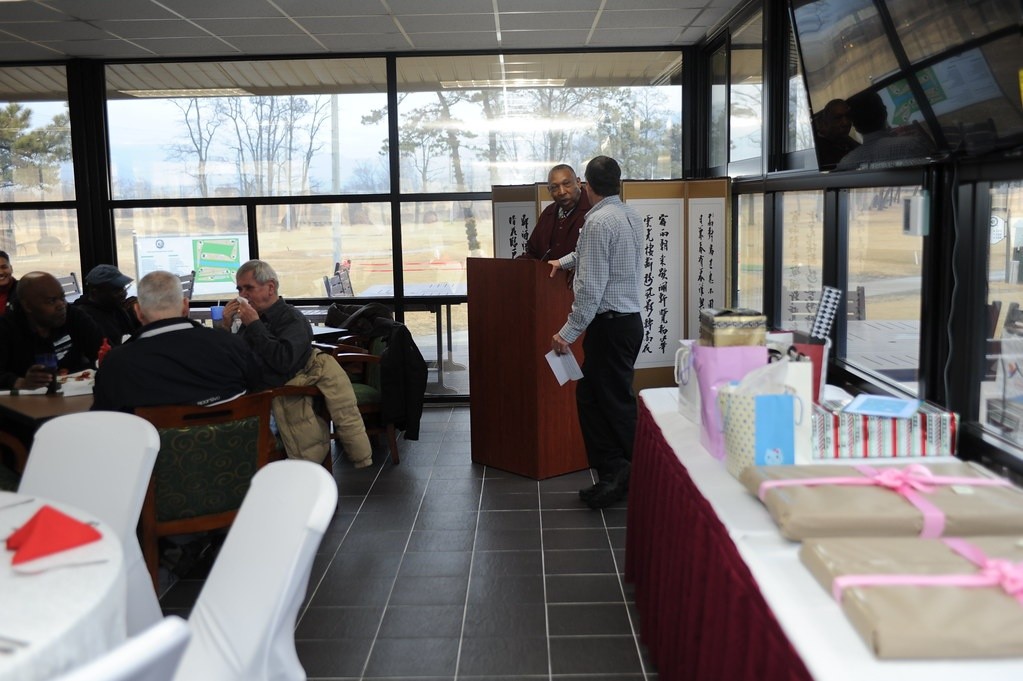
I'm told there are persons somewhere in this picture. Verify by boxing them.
[516,164,591,261]
[548,155,646,506]
[222,259,314,392]
[0,250,18,313]
[71,263,139,367]
[813,90,935,171]
[0,271,97,390]
[91,270,252,417]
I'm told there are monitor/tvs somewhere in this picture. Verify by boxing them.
[788,0,1023,173]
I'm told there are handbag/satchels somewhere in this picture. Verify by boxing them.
[673,336,813,481]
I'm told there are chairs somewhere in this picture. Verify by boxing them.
[0,259,406,681]
[56,259,354,299]
[984,302,1023,383]
[781,285,866,321]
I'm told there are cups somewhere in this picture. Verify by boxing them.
[211,306,224,320]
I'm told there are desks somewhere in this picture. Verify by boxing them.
[0,490,127,681]
[357,282,467,394]
[782,320,920,382]
[626,383,1023,681]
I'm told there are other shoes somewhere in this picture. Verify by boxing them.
[579,475,628,509]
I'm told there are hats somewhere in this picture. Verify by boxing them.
[85,264,135,289]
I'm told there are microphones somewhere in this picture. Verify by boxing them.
[541,249,552,261]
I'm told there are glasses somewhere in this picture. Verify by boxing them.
[102,284,132,298]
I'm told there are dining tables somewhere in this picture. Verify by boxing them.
[0,325,349,494]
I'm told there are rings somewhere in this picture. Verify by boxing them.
[237,309,240,312]
[556,349,561,352]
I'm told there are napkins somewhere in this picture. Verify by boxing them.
[7,504,102,564]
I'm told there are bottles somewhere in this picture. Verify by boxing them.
[98,338,112,366]
